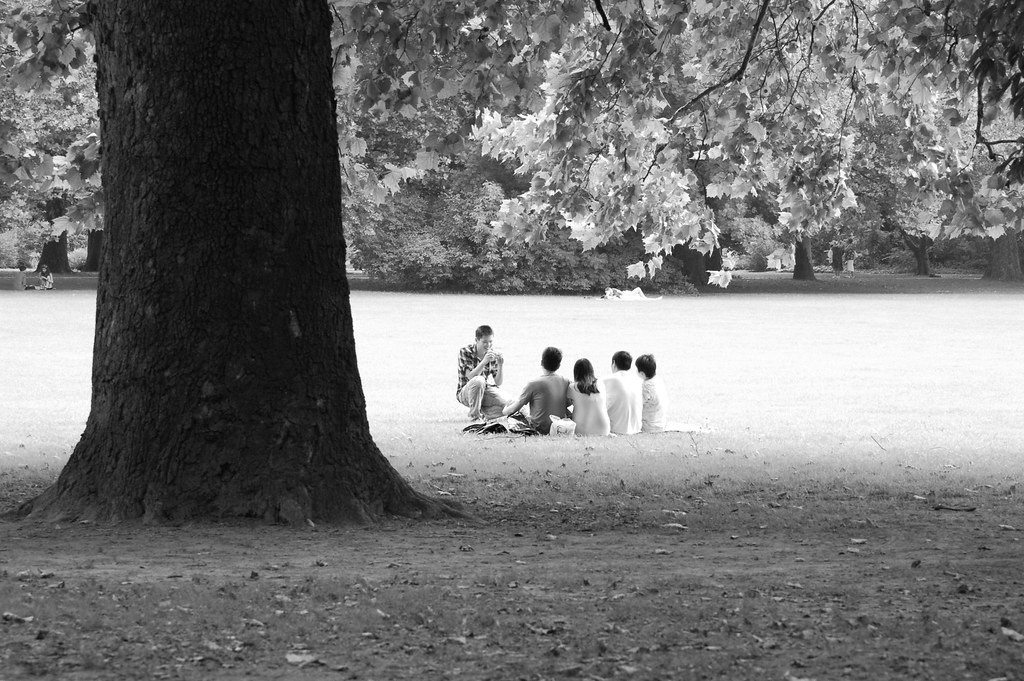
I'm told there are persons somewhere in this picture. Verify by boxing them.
[456,324,507,424]
[502,346,574,437]
[635,354,667,434]
[844,257,854,277]
[13,264,35,289]
[602,350,644,434]
[604,286,663,300]
[566,357,611,435]
[34,264,54,289]
